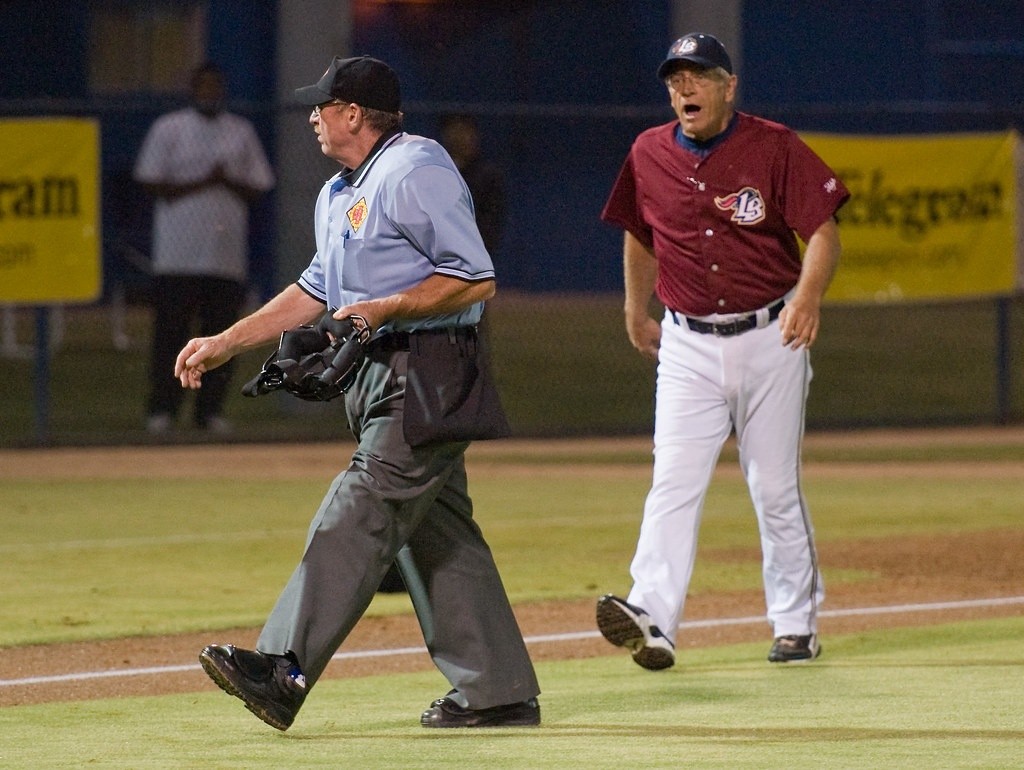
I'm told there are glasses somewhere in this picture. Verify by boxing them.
[312,101,347,113]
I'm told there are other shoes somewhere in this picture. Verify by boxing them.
[197,642,306,731]
[420,697,542,730]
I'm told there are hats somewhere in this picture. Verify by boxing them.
[656,32,732,78]
[294,52,403,114]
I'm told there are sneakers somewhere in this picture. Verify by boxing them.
[593,593,676,672]
[767,635,824,665]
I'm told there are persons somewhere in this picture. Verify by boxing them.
[131,67,276,436]
[597,33,852,670]
[436,106,509,258]
[174,55,541,732]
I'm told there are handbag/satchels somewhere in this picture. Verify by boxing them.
[402,328,513,447]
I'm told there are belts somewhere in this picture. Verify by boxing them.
[375,322,480,352]
[669,299,787,338]
